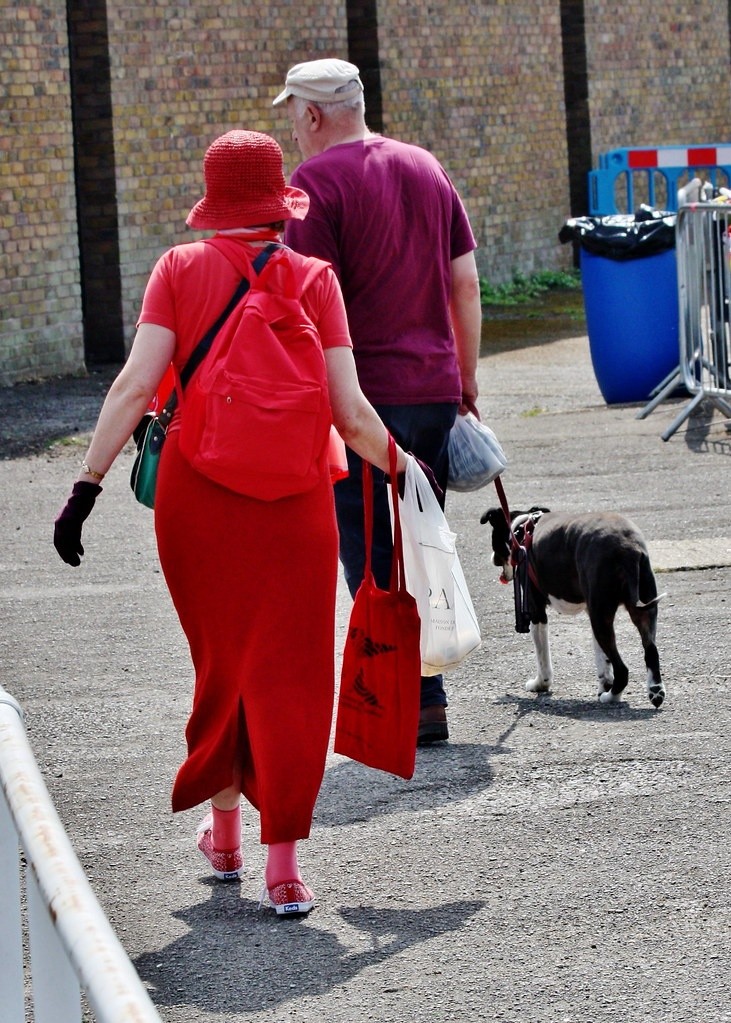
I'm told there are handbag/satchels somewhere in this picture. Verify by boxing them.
[448,405,507,494]
[129,408,166,509]
[387,452,483,679]
[334,444,421,783]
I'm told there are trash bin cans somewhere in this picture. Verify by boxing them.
[577,210,703,405]
[679,205,731,323]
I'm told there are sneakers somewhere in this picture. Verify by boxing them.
[257,880,315,915]
[195,813,244,880]
[416,706,450,741]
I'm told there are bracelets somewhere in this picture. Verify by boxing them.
[80,461,104,480]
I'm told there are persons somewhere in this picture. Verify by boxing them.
[272,58,482,745]
[53,128,445,916]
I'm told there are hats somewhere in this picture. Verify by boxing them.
[183,129,310,230]
[272,58,365,105]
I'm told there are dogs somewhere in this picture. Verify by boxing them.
[480,506,668,708]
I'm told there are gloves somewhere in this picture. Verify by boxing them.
[383,450,443,511]
[54,480,103,568]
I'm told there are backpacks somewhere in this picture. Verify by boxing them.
[176,238,335,501]
